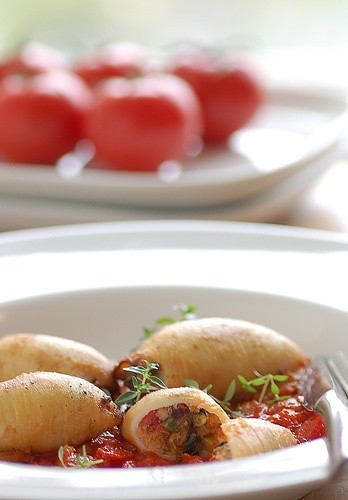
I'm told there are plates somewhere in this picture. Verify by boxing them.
[0,140,346,232]
[0,78,347,205]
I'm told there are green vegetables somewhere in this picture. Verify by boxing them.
[104,300,296,417]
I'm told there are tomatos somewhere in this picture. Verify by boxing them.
[0,40,264,173]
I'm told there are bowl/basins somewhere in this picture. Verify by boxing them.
[0,284,348,500]
